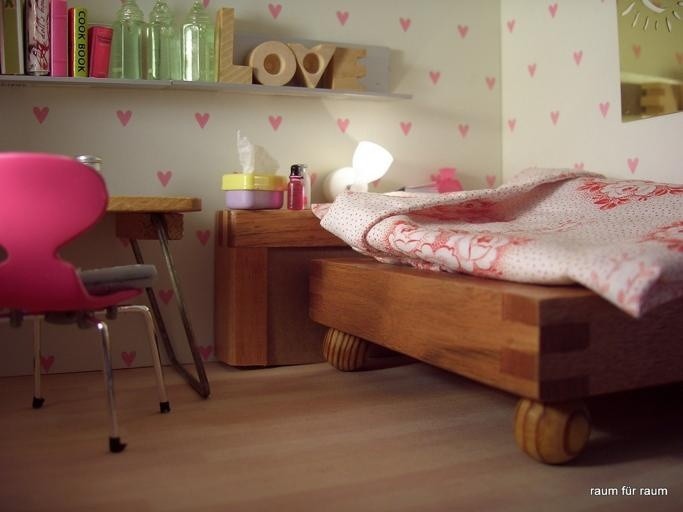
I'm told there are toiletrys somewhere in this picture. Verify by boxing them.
[286,164,305,210]
[300,163,310,209]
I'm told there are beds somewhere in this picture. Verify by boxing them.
[308,258,683,465]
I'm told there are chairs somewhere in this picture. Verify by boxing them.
[0,150,170,454]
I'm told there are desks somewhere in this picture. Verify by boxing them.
[107,197,211,399]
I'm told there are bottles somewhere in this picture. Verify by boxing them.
[106,1,145,80]
[300,162,311,211]
[179,0,220,82]
[143,1,181,81]
[285,164,306,212]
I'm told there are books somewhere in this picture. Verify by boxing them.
[0,0,112,77]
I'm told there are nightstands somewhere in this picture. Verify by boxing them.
[215,209,355,368]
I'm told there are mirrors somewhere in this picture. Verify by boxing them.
[617,0,683,124]
